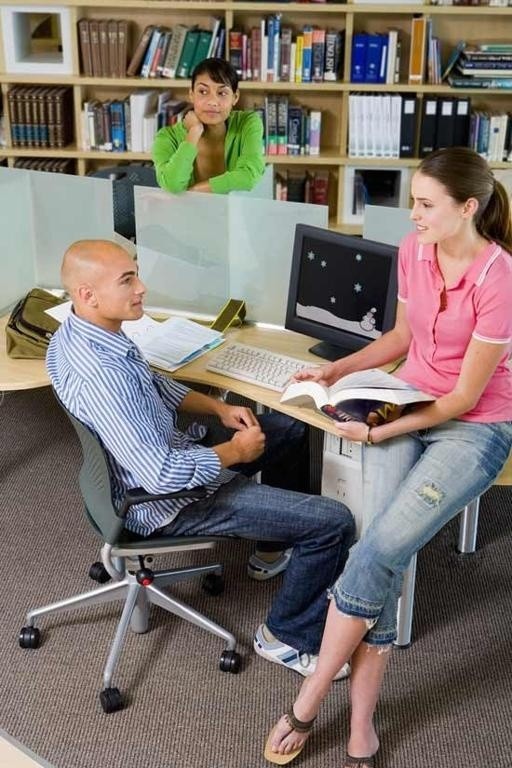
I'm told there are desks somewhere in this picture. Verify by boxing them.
[1,305,66,410]
[155,322,512,648]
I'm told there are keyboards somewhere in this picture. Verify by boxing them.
[206,341,318,393]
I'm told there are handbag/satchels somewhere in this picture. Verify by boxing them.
[5,285,73,360]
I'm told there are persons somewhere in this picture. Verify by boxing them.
[45,238,351,683]
[151,55,267,193]
[261,143,512,768]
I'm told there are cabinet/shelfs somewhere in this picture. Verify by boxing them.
[1,1,511,238]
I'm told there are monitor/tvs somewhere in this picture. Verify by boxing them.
[283,223,400,360]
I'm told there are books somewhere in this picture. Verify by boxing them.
[274,165,330,204]
[8,85,512,161]
[11,159,156,184]
[79,14,512,88]
[43,297,226,375]
[278,365,438,425]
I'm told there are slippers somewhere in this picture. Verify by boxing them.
[262,677,318,765]
[341,736,381,768]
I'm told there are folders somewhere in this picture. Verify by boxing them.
[348,92,416,159]
[418,96,471,159]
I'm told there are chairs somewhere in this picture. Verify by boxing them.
[15,378,246,709]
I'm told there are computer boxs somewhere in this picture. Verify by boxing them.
[323,429,419,541]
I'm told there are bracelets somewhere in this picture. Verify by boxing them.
[366,422,376,446]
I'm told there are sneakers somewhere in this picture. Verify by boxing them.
[254,621,352,680]
[248,546,293,580]
